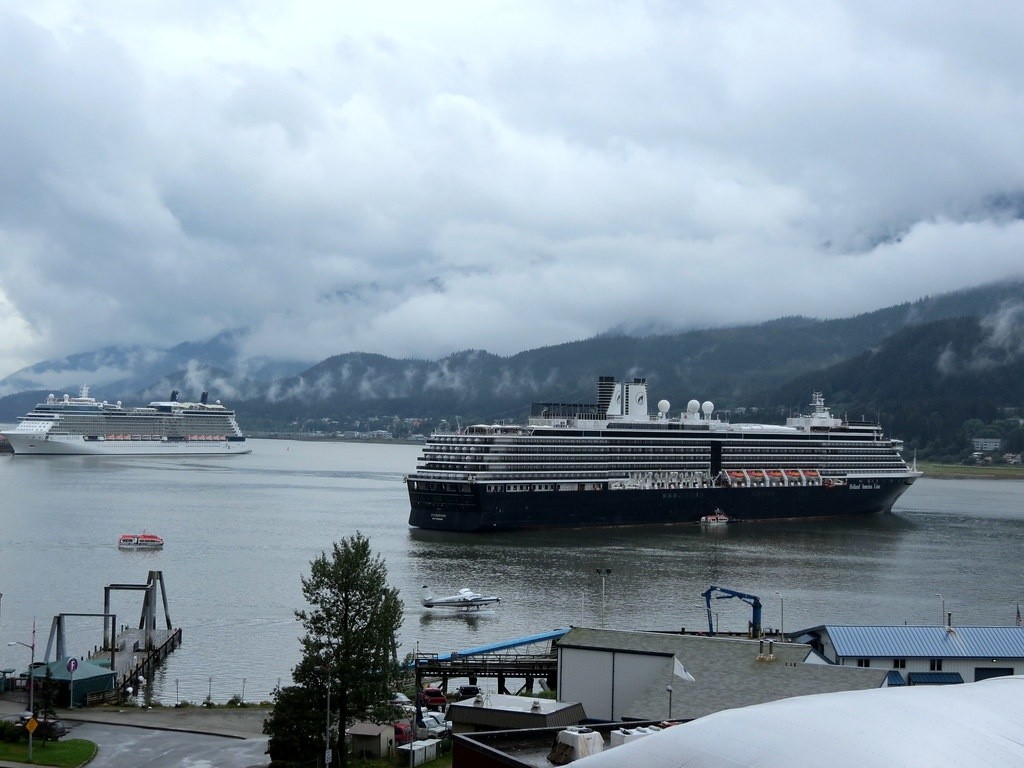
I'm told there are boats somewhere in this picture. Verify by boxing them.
[700,507,745,525]
[748,472,764,482]
[729,471,745,482]
[118,528,164,553]
[804,470,819,479]
[107,434,226,441]
[785,470,800,481]
[768,471,782,480]
[421,586,501,612]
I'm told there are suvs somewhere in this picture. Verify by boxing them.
[13,719,66,741]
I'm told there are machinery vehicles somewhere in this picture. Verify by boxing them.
[700,586,765,640]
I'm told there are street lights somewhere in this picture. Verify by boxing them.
[936,593,946,625]
[596,567,612,628]
[6,615,38,764]
[775,590,785,641]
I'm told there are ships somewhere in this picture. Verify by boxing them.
[0,391,253,455]
[403,376,924,530]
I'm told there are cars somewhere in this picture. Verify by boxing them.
[343,685,482,747]
